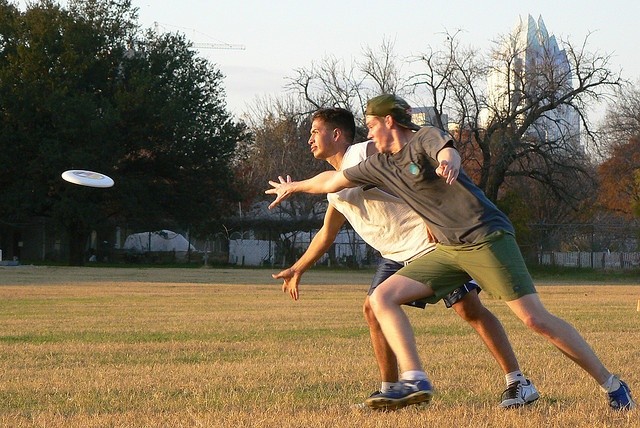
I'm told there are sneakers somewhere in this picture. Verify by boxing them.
[365,378,433,411]
[605,379,636,413]
[497,379,540,409]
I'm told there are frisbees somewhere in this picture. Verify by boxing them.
[61,170,114,188]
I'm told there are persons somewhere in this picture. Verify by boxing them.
[265,94,633,411]
[272,107,540,409]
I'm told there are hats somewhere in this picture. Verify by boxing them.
[363,94,421,131]
